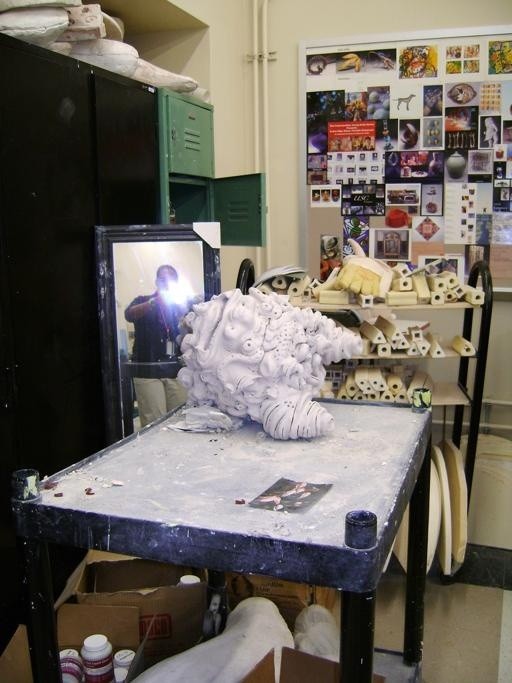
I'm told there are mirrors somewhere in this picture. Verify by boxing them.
[92,218,226,451]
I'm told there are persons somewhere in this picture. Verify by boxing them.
[123,265,193,428]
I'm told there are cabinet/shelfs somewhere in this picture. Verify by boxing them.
[1,31,272,666]
[232,253,495,586]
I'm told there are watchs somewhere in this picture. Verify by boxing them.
[149,296,158,308]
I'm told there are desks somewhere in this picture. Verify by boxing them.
[7,377,435,683]
